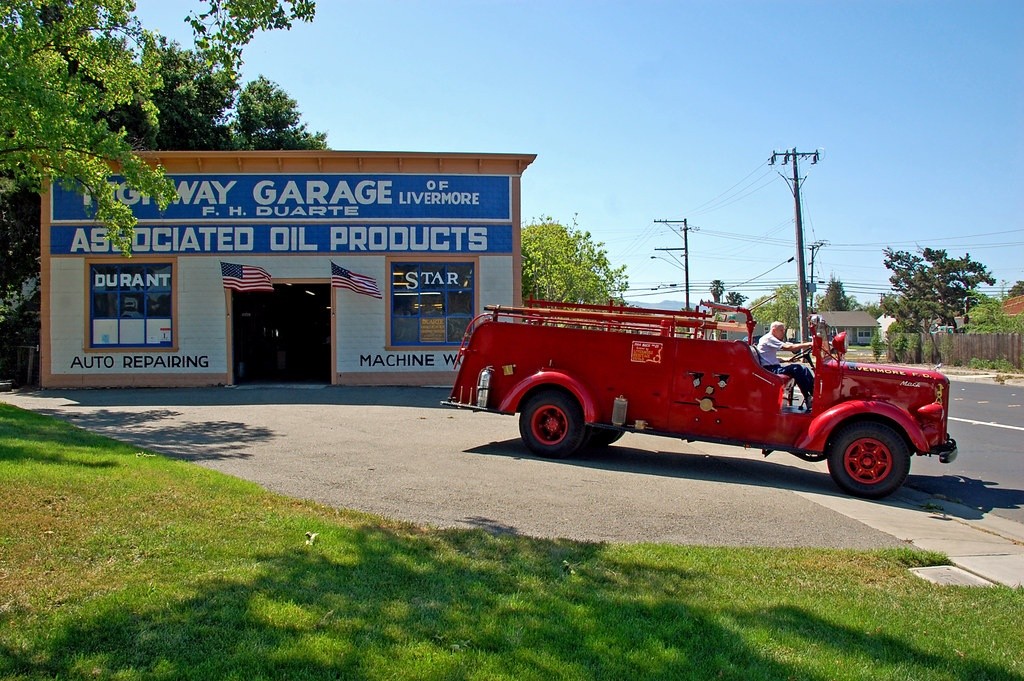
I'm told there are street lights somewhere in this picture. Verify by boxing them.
[651,255,690,337]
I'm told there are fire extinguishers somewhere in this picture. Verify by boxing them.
[475,366,495,409]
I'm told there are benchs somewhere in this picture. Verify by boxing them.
[750,345,797,389]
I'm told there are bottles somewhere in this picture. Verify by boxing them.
[611,395,628,427]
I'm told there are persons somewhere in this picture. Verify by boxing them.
[753,320,813,409]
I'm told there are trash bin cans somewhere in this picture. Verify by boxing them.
[753,336,762,345]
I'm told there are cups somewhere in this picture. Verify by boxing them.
[634,419,648,430]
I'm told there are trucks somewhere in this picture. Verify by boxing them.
[440,299,958,500]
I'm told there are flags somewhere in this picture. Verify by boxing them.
[330,260,384,300]
[220,262,274,291]
